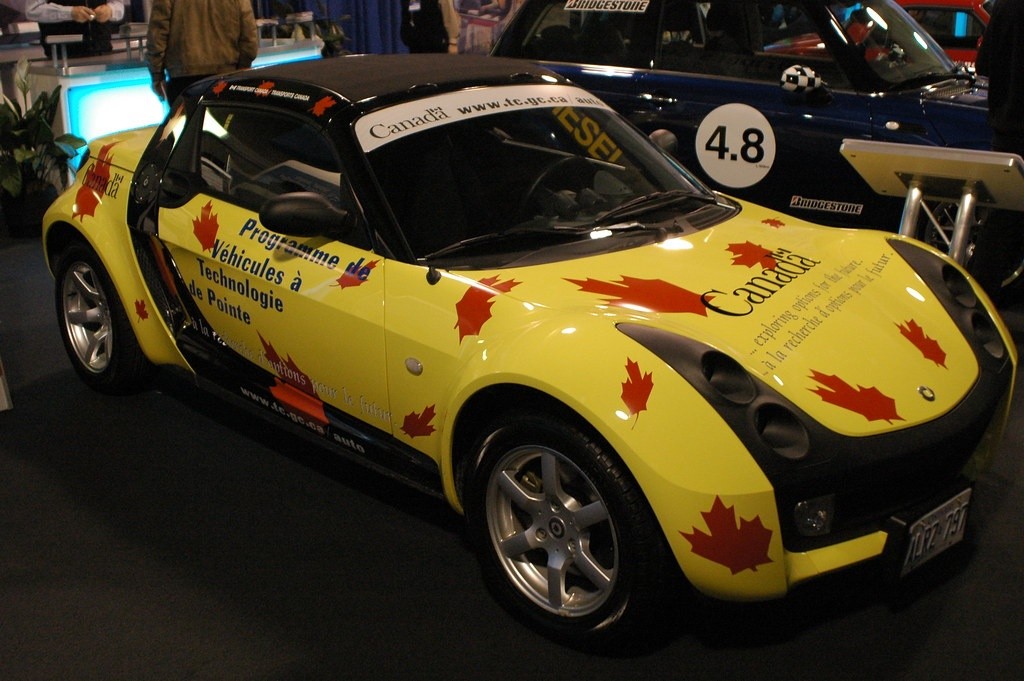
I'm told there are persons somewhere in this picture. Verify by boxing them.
[964,0,1024,290]
[400,0,459,100]
[455,0,512,54]
[145,0,259,109]
[25,0,125,60]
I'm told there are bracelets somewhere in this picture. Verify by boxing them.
[449,43,458,46]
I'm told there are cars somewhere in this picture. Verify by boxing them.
[483,0,993,265]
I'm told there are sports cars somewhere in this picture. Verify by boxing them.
[41,49,1020,647]
